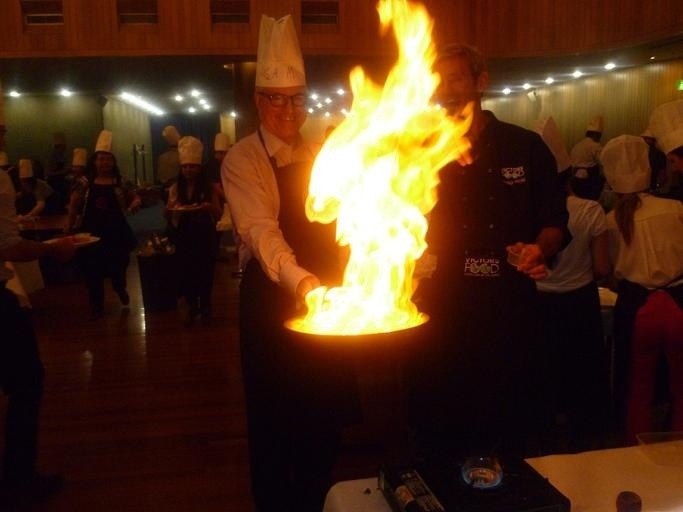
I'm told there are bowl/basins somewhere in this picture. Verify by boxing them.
[505,245,526,267]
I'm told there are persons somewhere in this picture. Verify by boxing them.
[404,42,573,471]
[532,99,682,457]
[222,14,361,512]
[1,125,231,329]
[0,118,77,512]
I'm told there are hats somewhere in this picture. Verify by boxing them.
[214,132,229,151]
[72,148,87,166]
[178,136,203,165]
[95,130,113,153]
[532,117,571,174]
[0,152,33,178]
[255,14,306,87]
[601,134,651,194]
[163,126,180,145]
[585,117,603,133]
[649,100,683,154]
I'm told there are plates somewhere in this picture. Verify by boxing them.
[42,235,101,248]
[169,207,201,211]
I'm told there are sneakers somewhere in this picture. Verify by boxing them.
[114,288,129,306]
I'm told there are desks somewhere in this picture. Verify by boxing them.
[320,439,683,512]
[17,214,81,233]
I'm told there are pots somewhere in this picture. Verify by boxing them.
[284,309,431,370]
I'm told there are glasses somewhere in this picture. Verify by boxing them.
[256,92,309,106]
